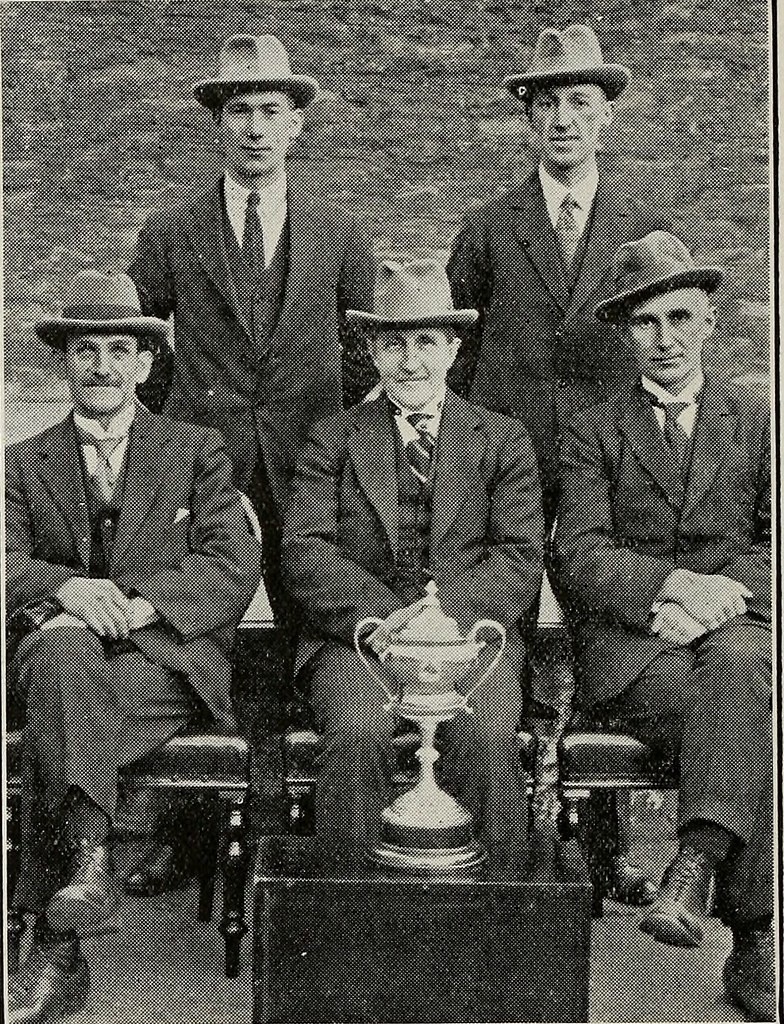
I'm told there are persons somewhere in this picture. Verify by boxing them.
[6,269,240,1010]
[285,254,545,845]
[553,230,775,1022]
[124,33,379,893]
[444,25,678,906]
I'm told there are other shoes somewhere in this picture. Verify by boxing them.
[723,948,777,1022]
[588,840,658,906]
[125,844,191,895]
[8,938,90,1023]
[638,850,710,950]
[46,863,121,938]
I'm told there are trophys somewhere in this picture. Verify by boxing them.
[353,579,506,874]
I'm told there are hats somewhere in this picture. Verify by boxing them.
[34,273,169,351]
[190,34,319,111]
[345,259,479,332]
[503,24,632,104]
[592,231,723,323]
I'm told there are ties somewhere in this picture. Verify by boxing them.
[387,400,444,487]
[553,193,582,275]
[641,380,706,478]
[241,191,265,274]
[72,426,132,505]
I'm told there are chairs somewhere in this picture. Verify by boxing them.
[5,483,683,922]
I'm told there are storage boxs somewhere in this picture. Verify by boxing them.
[253,827,592,1024]
